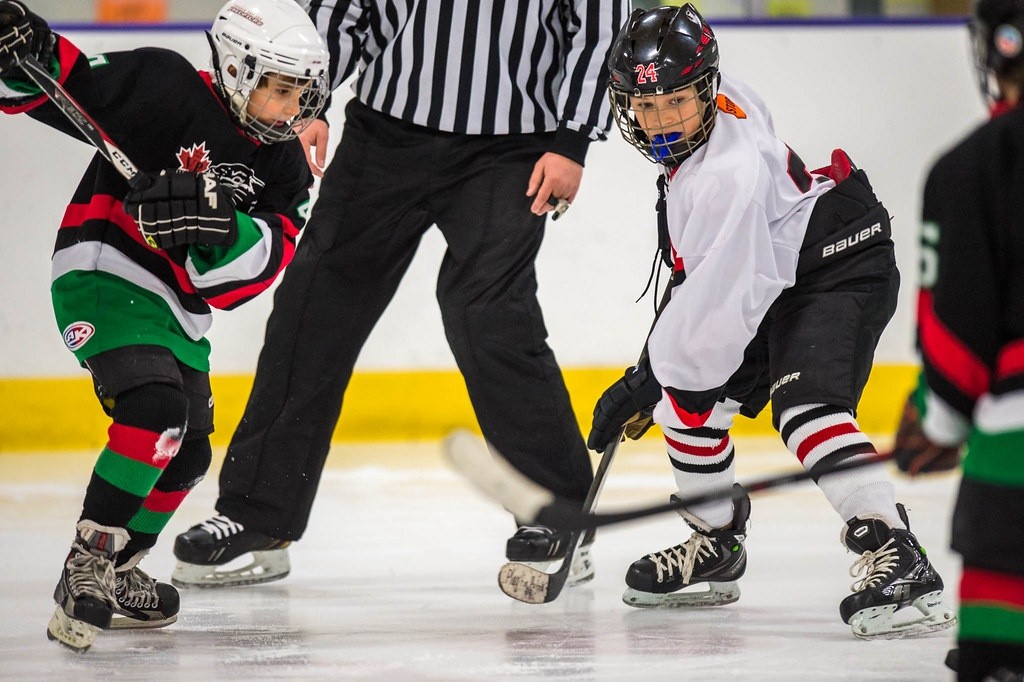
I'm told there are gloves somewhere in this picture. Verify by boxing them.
[887,391,965,478]
[0,0,57,81]
[122,170,239,248]
[586,356,664,454]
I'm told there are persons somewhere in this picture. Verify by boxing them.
[171,1,631,592]
[894,0,1024,682]
[588,4,956,643]
[0,0,330,655]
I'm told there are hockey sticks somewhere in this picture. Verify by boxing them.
[498,267,680,604]
[12,52,139,182]
[441,434,960,532]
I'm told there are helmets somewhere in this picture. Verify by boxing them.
[606,2,722,168]
[964,0,1024,103]
[204,0,331,145]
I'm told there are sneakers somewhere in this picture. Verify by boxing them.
[839,502,959,641]
[109,548,179,629]
[624,482,750,608]
[45,519,131,654]
[168,512,290,589]
[496,513,598,605]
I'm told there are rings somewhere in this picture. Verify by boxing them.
[547,194,570,215]
